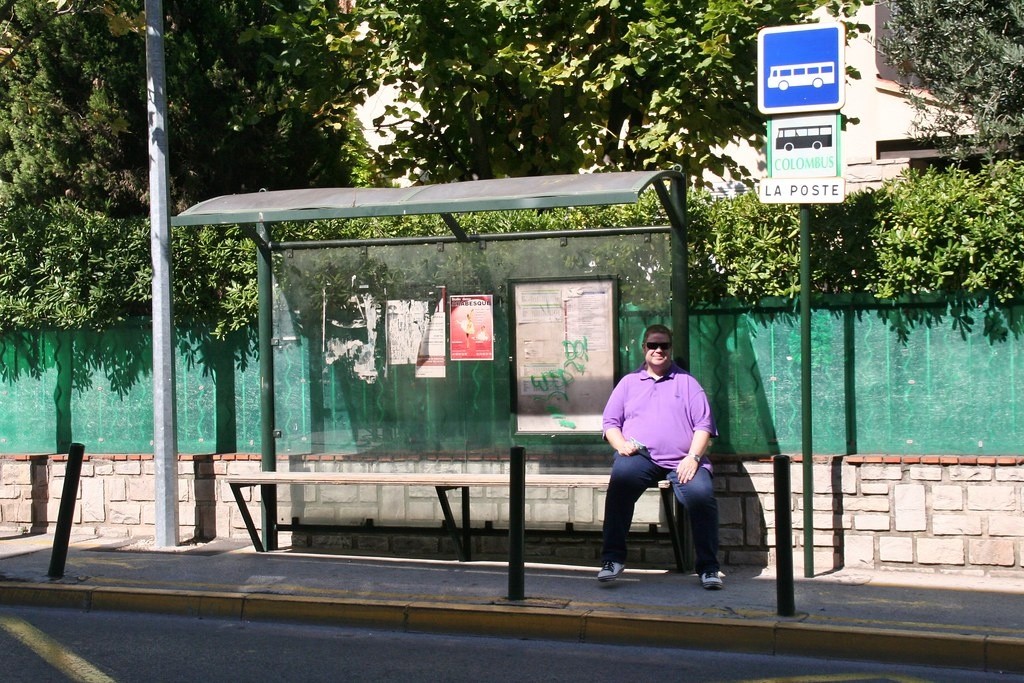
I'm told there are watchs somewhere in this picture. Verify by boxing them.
[688,453,700,462]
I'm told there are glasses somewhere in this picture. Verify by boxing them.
[644,342,671,350]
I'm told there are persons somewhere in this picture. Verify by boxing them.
[598,324,723,589]
[456,308,491,348]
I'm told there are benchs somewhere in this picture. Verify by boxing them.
[225,471,695,574]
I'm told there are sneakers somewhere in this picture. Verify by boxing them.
[598,561,624,582]
[698,571,723,589]
